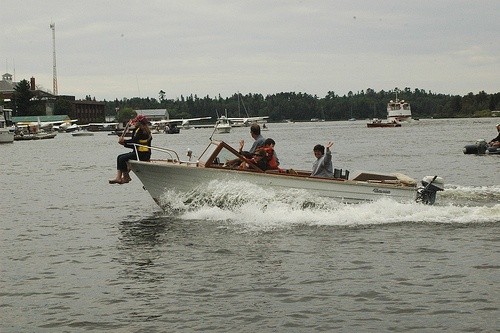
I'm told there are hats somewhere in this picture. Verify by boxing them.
[136,115,148,124]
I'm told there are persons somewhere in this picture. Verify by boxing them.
[489,125,500,148]
[311,142,334,178]
[109,115,151,184]
[239,124,279,170]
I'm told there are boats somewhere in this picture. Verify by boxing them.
[367,117,402,127]
[386,91,411,123]
[214,108,232,134]
[118,123,445,209]
[0,105,180,144]
